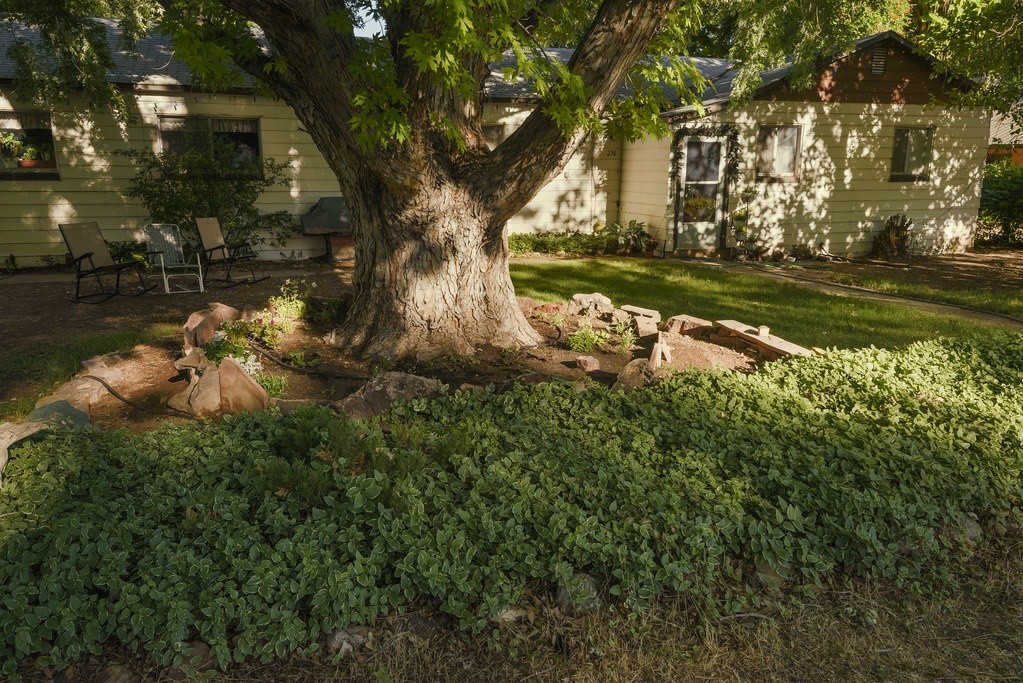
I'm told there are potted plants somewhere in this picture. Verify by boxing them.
[0,131,23,168]
[18,146,51,168]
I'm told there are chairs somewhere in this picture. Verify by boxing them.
[58,222,158,305]
[194,218,273,289]
[140,224,205,295]
